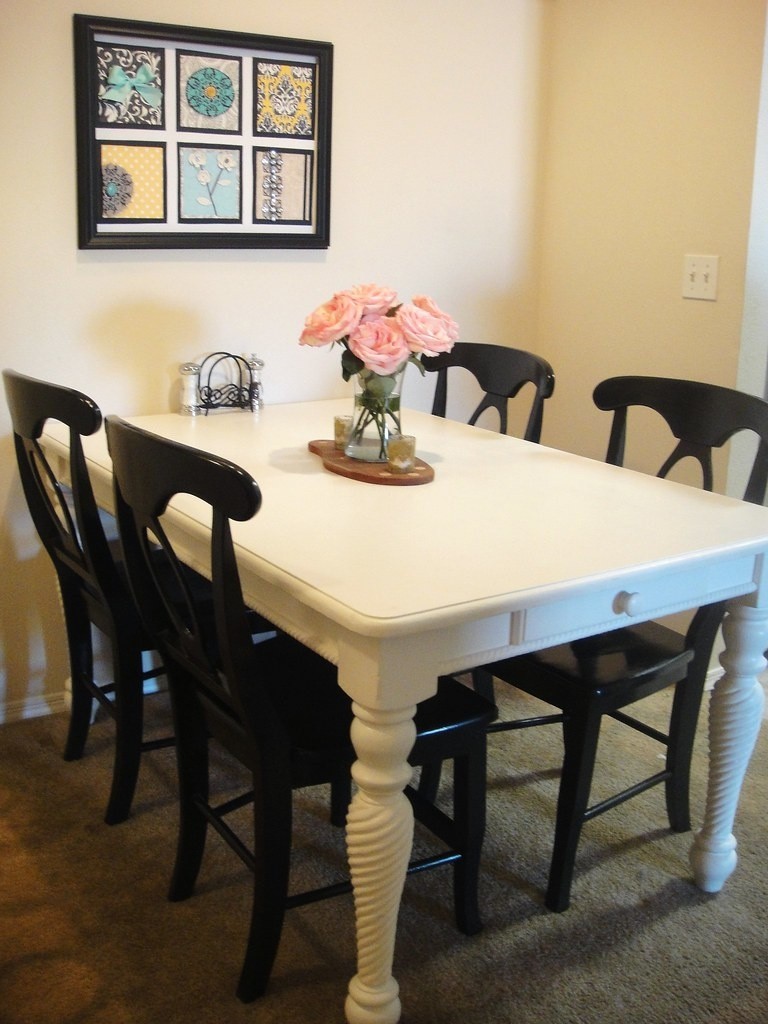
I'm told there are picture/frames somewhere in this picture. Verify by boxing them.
[73,11,334,249]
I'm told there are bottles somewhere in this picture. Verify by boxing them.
[179,362,201,416]
[245,357,263,412]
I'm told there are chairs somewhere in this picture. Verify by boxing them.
[468,376,768,910]
[421,339,556,718]
[102,418,487,1001]
[1,369,286,827]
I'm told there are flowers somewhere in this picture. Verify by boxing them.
[299,282,459,460]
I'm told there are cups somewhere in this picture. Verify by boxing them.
[388,435,416,473]
[335,415,353,450]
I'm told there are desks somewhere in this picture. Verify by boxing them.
[32,393,768,1023]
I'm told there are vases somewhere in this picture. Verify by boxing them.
[345,363,406,463]
